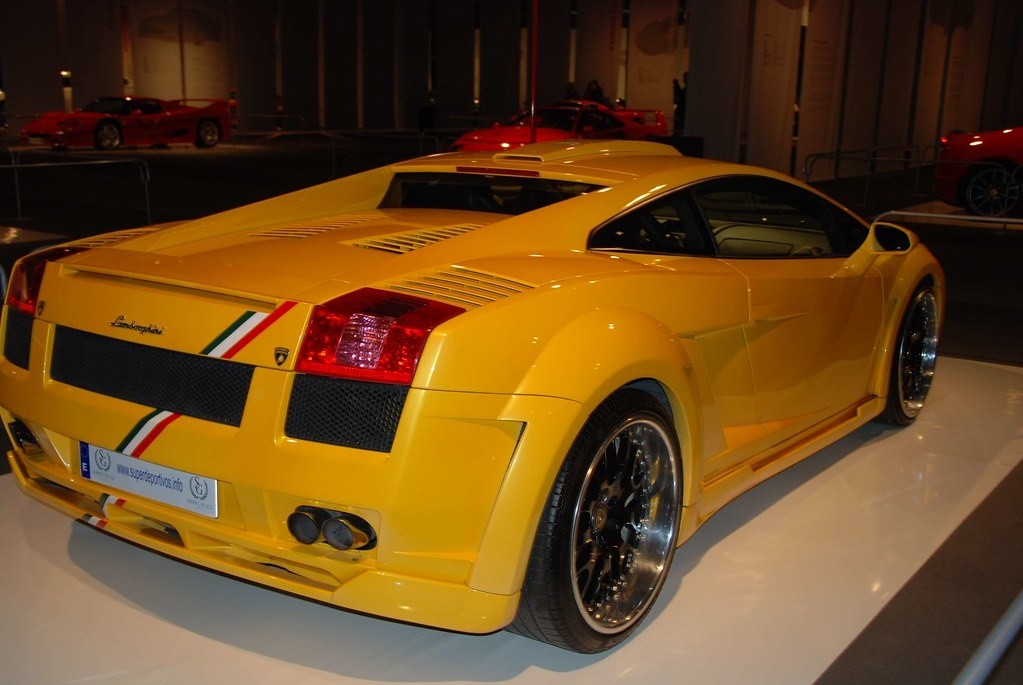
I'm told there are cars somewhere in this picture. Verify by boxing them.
[0,139,948,652]
[937,126,1023,218]
[22,93,232,151]
[449,98,667,155]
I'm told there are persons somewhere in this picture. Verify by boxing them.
[584,80,603,103]
[672,71,688,136]
[567,82,580,107]
